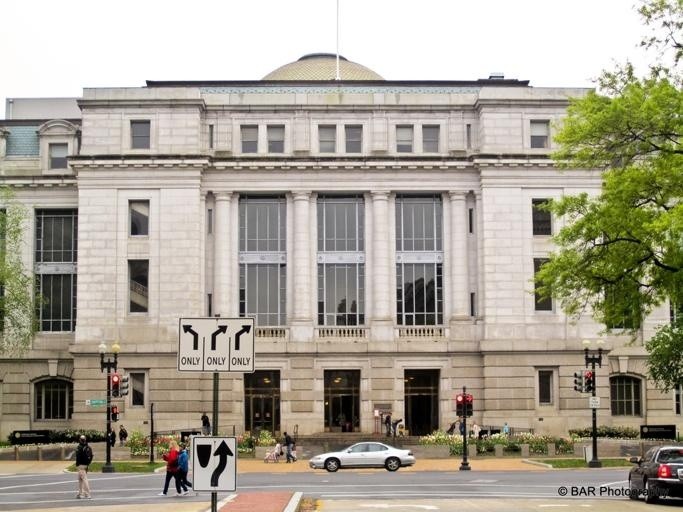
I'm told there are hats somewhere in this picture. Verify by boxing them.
[178,442,185,448]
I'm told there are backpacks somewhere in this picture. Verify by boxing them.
[82,445,92,464]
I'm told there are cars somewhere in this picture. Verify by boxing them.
[627,445,682,505]
[309,441,415,473]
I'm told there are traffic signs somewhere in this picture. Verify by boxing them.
[193,436,236,492]
[178,317,254,373]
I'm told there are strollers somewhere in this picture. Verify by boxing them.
[263,442,284,464]
[445,419,457,436]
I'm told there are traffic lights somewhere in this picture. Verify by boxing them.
[455,393,472,416]
[111,406,118,422]
[110,373,130,399]
[572,371,593,393]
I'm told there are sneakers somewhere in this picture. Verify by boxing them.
[77,494,91,498]
[157,492,167,496]
[183,491,189,495]
[176,494,181,496]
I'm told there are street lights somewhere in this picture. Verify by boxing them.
[98,343,122,473]
[581,337,605,468]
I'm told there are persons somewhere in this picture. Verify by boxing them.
[281,430,295,463]
[459,416,464,435]
[472,420,482,439]
[393,418,402,434]
[384,413,392,436]
[501,422,510,434]
[75,435,92,499]
[157,440,189,496]
[119,425,128,447]
[175,442,192,495]
[110,428,116,447]
[201,412,210,436]
